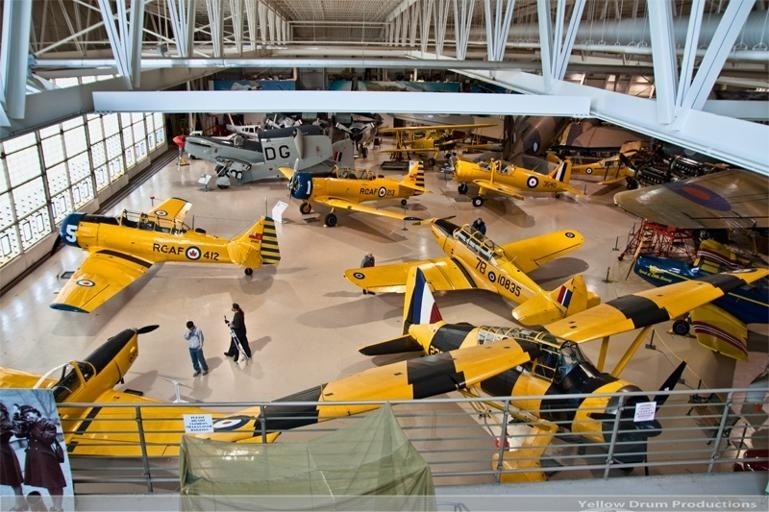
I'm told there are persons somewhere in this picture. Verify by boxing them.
[224,300,253,358]
[184,320,208,378]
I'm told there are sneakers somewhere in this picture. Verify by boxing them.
[192,371,208,376]
[224,352,232,357]
